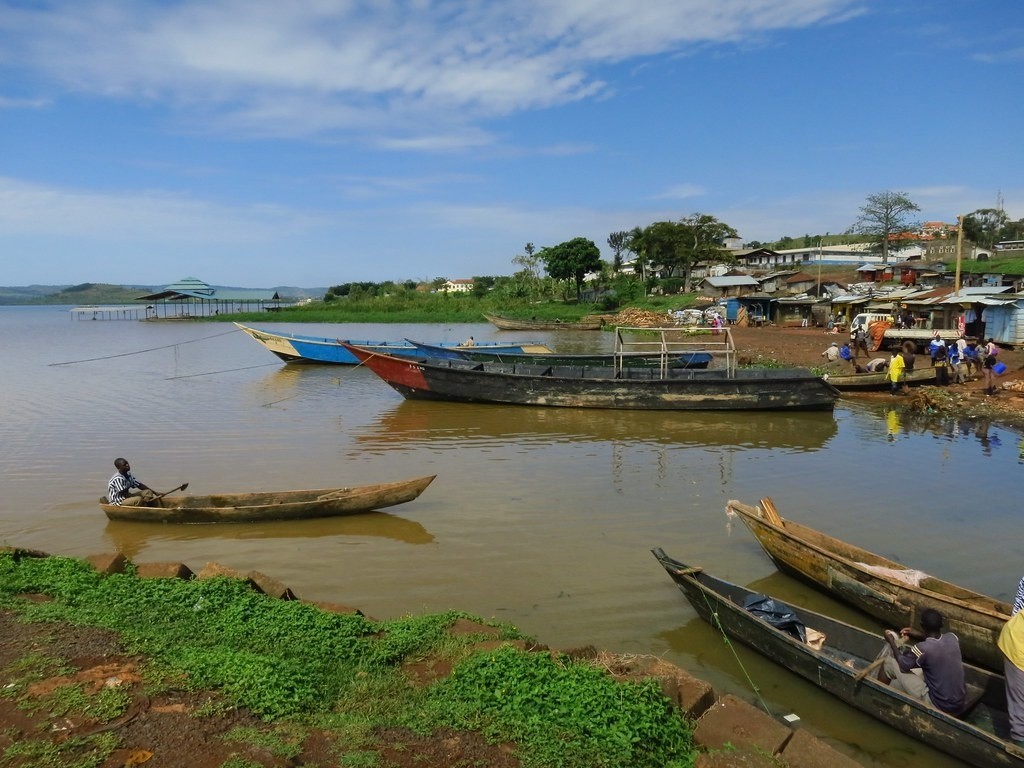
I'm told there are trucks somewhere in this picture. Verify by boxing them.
[849,312,964,355]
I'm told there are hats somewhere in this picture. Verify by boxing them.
[832,342,838,346]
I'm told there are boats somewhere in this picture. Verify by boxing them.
[99,473,438,523]
[403,337,714,370]
[480,312,603,330]
[650,543,1024,768]
[726,497,1015,674]
[231,320,559,367]
[335,322,844,412]
[818,356,986,385]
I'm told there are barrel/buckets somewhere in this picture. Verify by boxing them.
[992,362,1006,374]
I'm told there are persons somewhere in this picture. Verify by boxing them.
[876,607,965,718]
[463,336,474,347]
[600,317,606,328]
[821,300,997,396]
[996,576,1024,748]
[711,316,723,336]
[107,457,165,507]
[881,403,1024,465]
[801,309,808,327]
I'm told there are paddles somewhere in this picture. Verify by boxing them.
[148,482,189,502]
